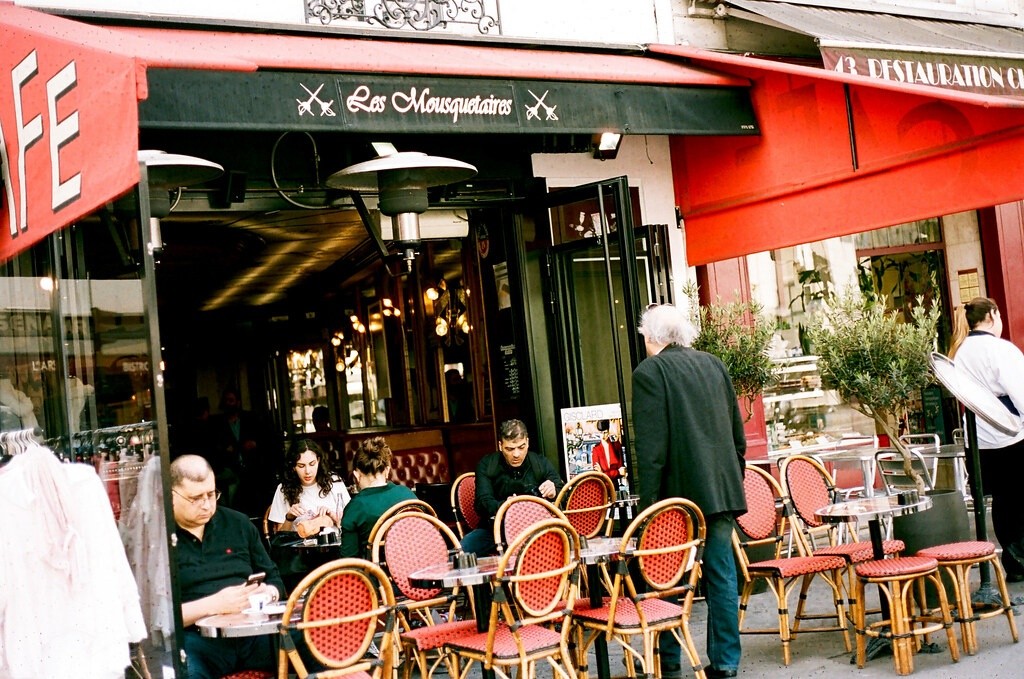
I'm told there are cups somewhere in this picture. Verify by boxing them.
[248,593,273,621]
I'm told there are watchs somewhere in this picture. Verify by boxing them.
[268,586,276,603]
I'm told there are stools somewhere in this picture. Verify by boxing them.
[855,558,960,675]
[914,543,1018,656]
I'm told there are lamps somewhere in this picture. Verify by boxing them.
[594,132,623,159]
[327,143,480,270]
[114,148,223,249]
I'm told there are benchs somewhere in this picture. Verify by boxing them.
[339,419,495,483]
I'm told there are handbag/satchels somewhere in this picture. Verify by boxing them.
[295,513,334,537]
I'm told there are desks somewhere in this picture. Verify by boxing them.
[918,442,1003,566]
[196,598,305,679]
[813,496,944,665]
[410,555,519,679]
[290,534,344,550]
[563,536,639,678]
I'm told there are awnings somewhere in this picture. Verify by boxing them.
[0,0,1024,262]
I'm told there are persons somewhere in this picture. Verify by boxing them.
[340,437,426,597]
[948,297,1024,582]
[631,303,748,679]
[311,407,342,445]
[592,419,626,496]
[451,418,565,616]
[169,455,328,679]
[268,438,351,536]
[193,390,257,507]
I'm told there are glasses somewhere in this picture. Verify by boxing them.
[646,303,672,311]
[172,487,221,508]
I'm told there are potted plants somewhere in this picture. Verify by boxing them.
[804,269,971,607]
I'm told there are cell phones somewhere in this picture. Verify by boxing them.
[245,573,265,587]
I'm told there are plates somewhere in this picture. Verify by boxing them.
[242,605,287,615]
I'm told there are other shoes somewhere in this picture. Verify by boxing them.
[1006,572,1024,583]
[1006,544,1024,567]
[704,665,737,679]
[427,660,448,674]
[624,655,681,678]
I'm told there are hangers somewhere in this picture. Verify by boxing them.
[0,428,48,466]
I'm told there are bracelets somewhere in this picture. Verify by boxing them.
[286,514,297,521]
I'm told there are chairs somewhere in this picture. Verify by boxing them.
[263,456,904,678]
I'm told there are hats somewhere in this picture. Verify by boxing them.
[597,419,610,431]
